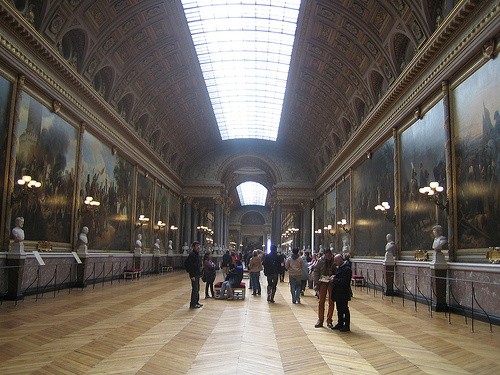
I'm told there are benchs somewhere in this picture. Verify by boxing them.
[351,274,365,290]
[124,268,141,279]
[214,282,246,299]
[162,265,173,273]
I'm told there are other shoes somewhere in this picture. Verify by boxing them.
[340,327,350,332]
[297,297,300,303]
[327,322,333,328]
[212,292,214,298]
[227,296,235,300]
[293,300,296,304]
[300,290,304,296]
[205,291,209,297]
[315,322,323,327]
[216,296,224,299]
[191,303,203,308]
[331,325,344,330]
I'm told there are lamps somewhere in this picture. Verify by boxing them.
[418,182,449,213]
[135,215,149,231]
[11,175,41,209]
[154,221,166,233]
[337,218,351,234]
[324,224,335,237]
[77,197,101,219]
[374,201,396,225]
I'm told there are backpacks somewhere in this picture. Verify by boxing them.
[200,268,206,282]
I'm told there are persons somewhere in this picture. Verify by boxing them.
[78,226,89,245]
[264,244,281,302]
[136,234,142,247]
[285,247,304,304]
[168,240,173,249]
[216,250,243,300]
[432,225,448,252]
[244,248,265,296]
[154,239,160,249]
[305,240,350,252]
[12,217,25,243]
[314,248,339,328]
[182,242,189,251]
[189,241,203,309]
[385,234,395,253]
[344,255,353,301]
[328,254,353,332]
[277,252,288,283]
[203,251,216,298]
[299,252,327,299]
